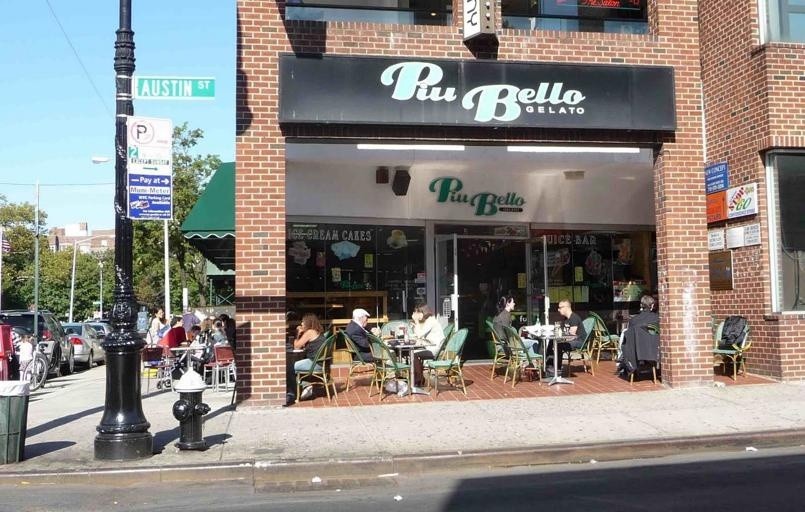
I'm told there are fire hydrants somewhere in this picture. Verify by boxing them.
[170,362,213,452]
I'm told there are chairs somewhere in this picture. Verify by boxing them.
[141,342,237,394]
[713,316,753,381]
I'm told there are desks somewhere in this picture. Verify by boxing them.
[287,349,304,376]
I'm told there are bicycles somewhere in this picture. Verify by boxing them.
[22,339,53,392]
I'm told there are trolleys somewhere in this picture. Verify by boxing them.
[138,328,174,392]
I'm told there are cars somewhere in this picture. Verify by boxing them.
[58,319,114,371]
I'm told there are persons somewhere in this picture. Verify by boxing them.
[621,294,659,376]
[157,314,236,363]
[493,294,540,359]
[18,334,33,381]
[546,299,588,374]
[289,312,327,400]
[345,308,399,378]
[146,308,165,348]
[412,303,444,387]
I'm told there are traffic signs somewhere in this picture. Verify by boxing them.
[131,77,215,99]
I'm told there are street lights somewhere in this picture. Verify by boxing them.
[68,235,117,327]
[89,154,174,325]
[94,256,107,319]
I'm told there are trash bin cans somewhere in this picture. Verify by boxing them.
[0,380,29,464]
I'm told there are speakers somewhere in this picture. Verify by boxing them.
[376,167,389,183]
[392,170,411,196]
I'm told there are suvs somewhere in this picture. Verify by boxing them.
[3,307,76,378]
